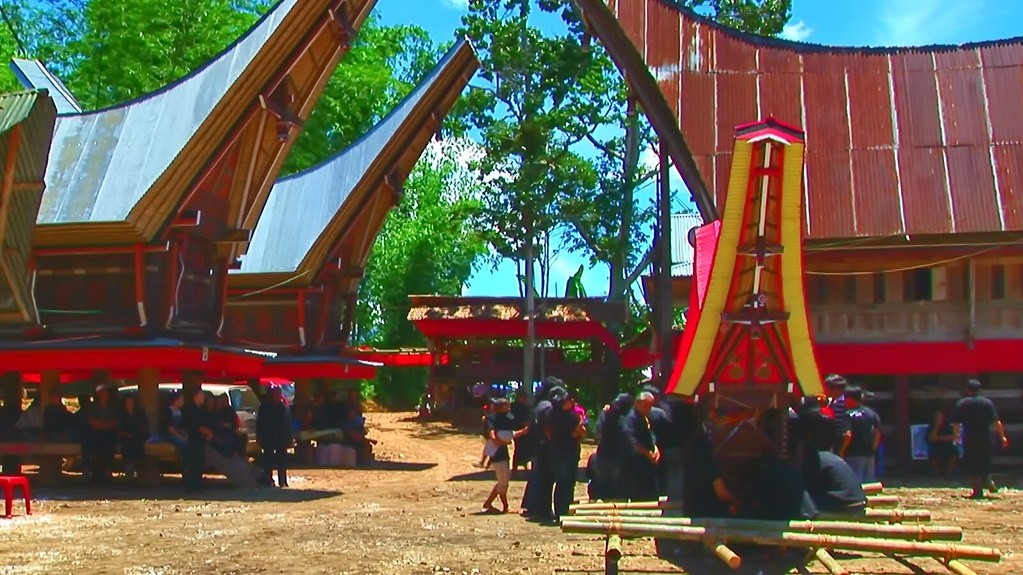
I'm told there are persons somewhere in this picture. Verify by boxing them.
[0,377,378,496]
[476,374,1010,575]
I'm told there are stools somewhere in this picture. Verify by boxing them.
[0,475,32,519]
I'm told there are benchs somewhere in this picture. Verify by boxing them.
[0,441,261,482]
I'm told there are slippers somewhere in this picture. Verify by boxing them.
[501,508,519,514]
[480,506,500,514]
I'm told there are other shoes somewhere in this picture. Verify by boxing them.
[988,481,998,493]
[963,494,984,499]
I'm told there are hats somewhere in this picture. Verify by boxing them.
[965,379,981,391]
[96,383,107,392]
